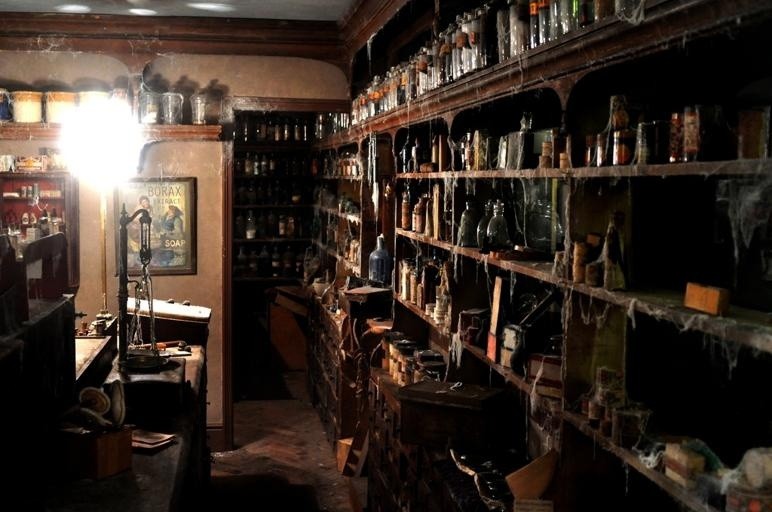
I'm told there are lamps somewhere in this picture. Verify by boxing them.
[58,93,150,425]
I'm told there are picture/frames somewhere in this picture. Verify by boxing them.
[113,176,199,277]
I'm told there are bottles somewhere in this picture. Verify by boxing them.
[350,0,489,125]
[315,212,360,266]
[495,0,639,65]
[604,93,626,165]
[602,211,627,293]
[19,182,40,197]
[458,130,486,171]
[571,231,601,287]
[398,190,434,239]
[401,134,412,172]
[234,210,304,239]
[582,132,608,166]
[303,247,314,283]
[518,110,535,170]
[236,111,327,141]
[323,151,359,176]
[234,244,295,278]
[412,138,424,172]
[429,122,438,172]
[475,201,510,251]
[38,207,59,236]
[234,153,312,175]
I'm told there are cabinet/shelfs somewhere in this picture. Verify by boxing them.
[1,168,83,299]
[1,295,116,505]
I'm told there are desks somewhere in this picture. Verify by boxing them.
[4,339,205,510]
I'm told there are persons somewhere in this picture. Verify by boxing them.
[128,194,155,224]
[157,205,184,264]
